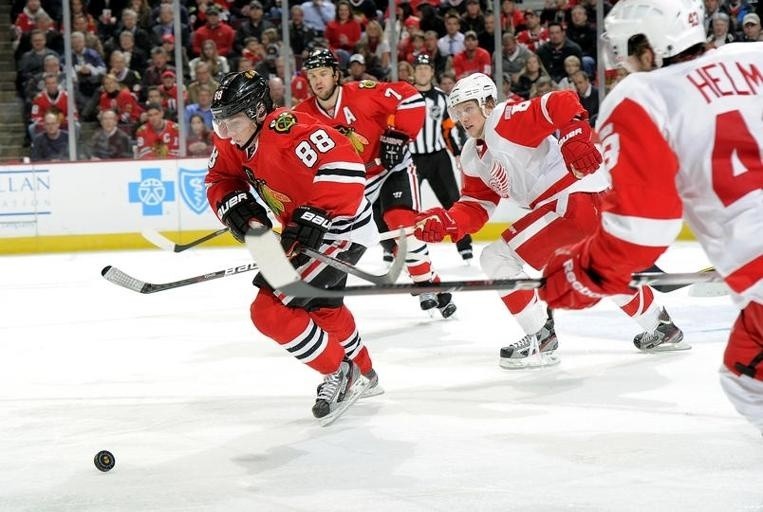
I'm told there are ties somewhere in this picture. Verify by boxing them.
[448,39,455,55]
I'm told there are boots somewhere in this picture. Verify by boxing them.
[501,306,558,358]
[634,305,684,350]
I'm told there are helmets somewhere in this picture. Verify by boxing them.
[446,72,497,125]
[210,69,274,140]
[597,1,708,72]
[302,49,341,70]
[412,53,435,69]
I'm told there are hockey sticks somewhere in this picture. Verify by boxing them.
[244,227,725,297]
[101,216,443,294]
[142,158,383,252]
[641,263,717,293]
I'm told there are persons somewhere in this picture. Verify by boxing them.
[537,0,763,431]
[0,1,763,163]
[382,53,474,268]
[291,48,459,321]
[202,70,387,429]
[412,71,694,368]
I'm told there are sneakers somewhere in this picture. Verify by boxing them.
[461,248,473,260]
[311,354,379,419]
[383,249,394,264]
[421,289,456,318]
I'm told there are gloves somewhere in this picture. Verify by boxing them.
[217,189,273,244]
[408,206,456,245]
[379,125,411,172]
[538,237,607,310]
[281,204,332,259]
[556,121,601,180]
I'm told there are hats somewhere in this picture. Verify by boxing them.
[522,6,537,19]
[265,43,280,61]
[162,69,176,78]
[405,16,419,27]
[205,5,220,16]
[248,1,263,9]
[161,33,176,44]
[742,13,761,27]
[464,29,479,41]
[350,54,366,66]
